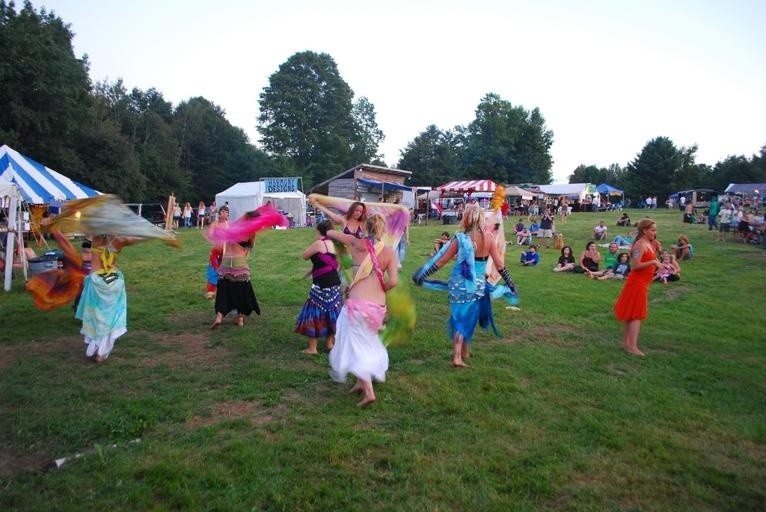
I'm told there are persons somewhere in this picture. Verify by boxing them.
[326,211,397,407]
[195,200,206,230]
[592,221,608,241]
[431,232,456,260]
[501,199,510,220]
[684,194,766,244]
[513,197,538,217]
[516,227,532,246]
[173,203,182,228]
[296,220,345,355]
[512,219,524,234]
[637,193,658,209]
[591,253,631,281]
[613,218,665,356]
[592,196,597,212]
[74,234,157,362]
[573,241,600,273]
[652,255,677,286]
[520,245,540,267]
[310,197,367,295]
[182,202,193,228]
[672,235,694,262]
[659,249,681,282]
[586,242,619,278]
[528,217,539,235]
[546,196,569,222]
[413,203,518,368]
[614,230,635,246]
[538,206,553,248]
[210,200,271,330]
[397,223,409,268]
[616,213,630,226]
[204,206,229,299]
[72,233,92,313]
[219,202,229,208]
[483,210,504,283]
[553,246,576,272]
[207,202,218,223]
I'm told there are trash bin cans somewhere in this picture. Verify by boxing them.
[27,256,59,274]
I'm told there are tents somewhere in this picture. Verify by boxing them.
[590,184,624,210]
[359,178,412,204]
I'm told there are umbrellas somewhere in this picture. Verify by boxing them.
[725,183,766,199]
[0,144,106,291]
